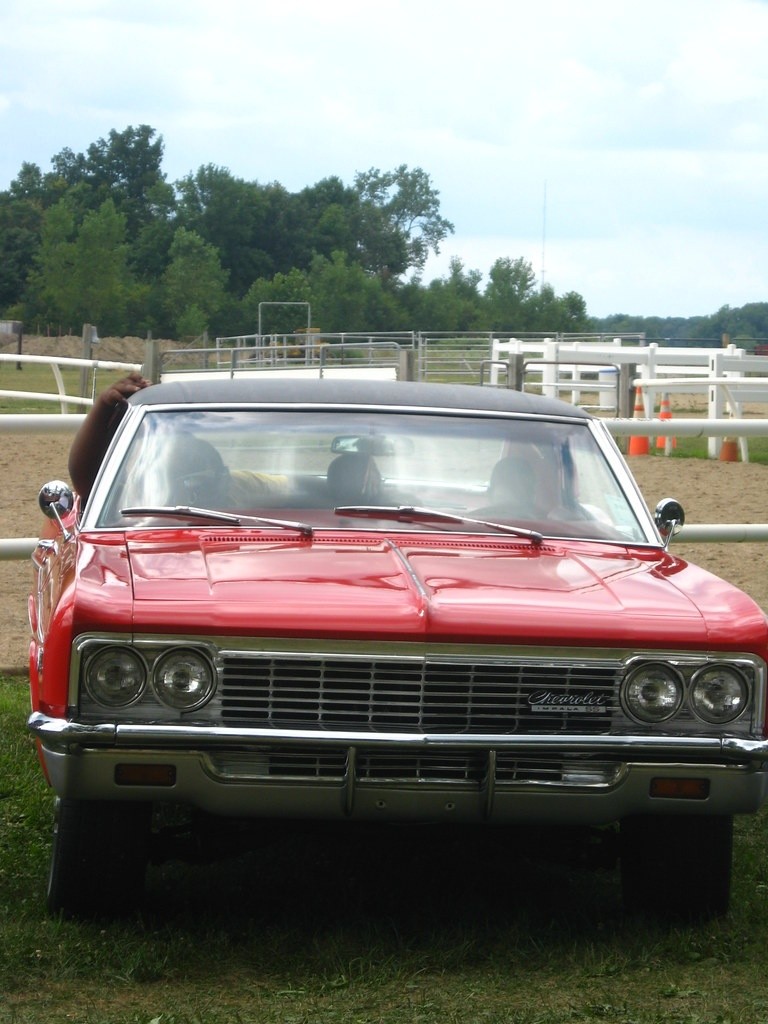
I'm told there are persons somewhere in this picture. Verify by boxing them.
[485,457,535,504]
[68,372,422,509]
[326,453,381,506]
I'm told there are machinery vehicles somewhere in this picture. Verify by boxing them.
[270,326,329,359]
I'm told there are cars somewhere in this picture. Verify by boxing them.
[22,373,768,924]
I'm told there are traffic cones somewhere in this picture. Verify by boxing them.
[716,409,742,463]
[627,383,656,458]
[655,392,678,450]
